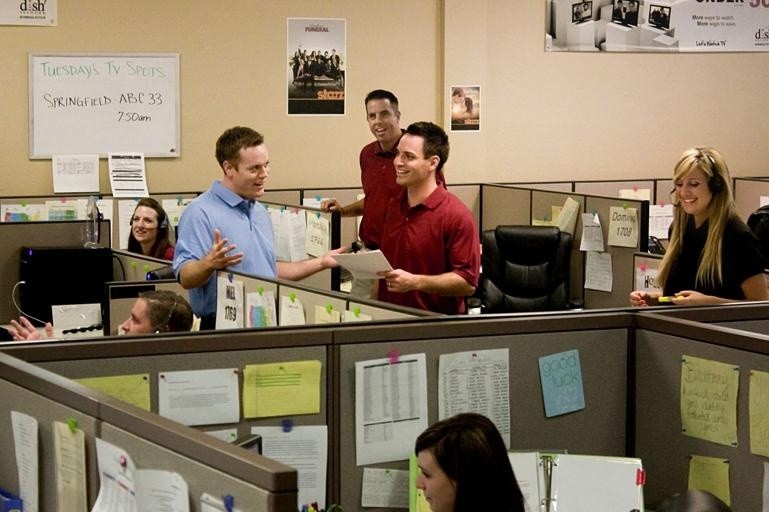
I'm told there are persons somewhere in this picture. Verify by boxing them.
[614,1,626,22]
[582,4,592,18]
[628,145,769,306]
[411,412,527,512]
[290,47,345,94]
[7,289,195,342]
[373,122,482,316]
[623,2,636,25]
[173,127,348,333]
[575,8,582,20]
[127,197,175,261]
[451,89,465,120]
[322,89,449,300]
[461,97,473,121]
[654,8,668,27]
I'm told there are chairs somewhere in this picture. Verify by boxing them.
[746,205,769,274]
[630,489,732,512]
[468,225,586,312]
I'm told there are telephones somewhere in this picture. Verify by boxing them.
[648,236,665,255]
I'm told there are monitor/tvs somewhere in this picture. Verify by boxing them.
[647,4,671,31]
[612,0,640,28]
[230,434,262,456]
[632,252,664,291]
[572,1,592,23]
[102,278,190,335]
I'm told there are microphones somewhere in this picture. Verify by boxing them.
[142,227,158,229]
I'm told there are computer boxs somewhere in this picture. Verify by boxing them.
[19,245,113,326]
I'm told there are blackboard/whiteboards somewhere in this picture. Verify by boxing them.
[29,52,182,160]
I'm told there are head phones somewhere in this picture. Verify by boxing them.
[707,154,724,193]
[159,212,168,233]
[152,298,177,334]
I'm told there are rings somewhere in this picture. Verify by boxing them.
[389,281,391,287]
